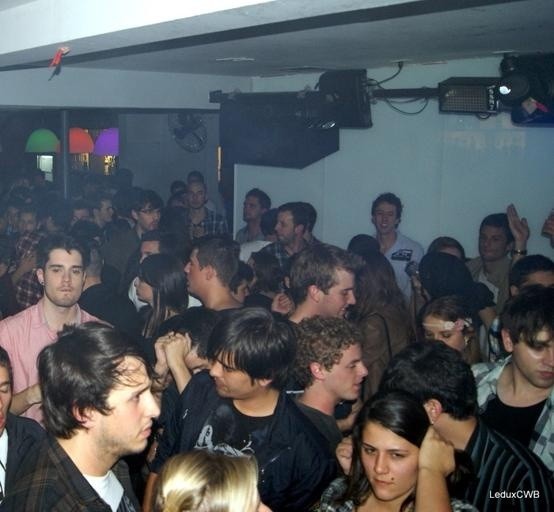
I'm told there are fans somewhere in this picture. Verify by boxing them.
[165,111,207,153]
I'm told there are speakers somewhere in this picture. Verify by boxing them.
[219,92,339,169]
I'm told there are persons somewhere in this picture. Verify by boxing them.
[0,159,553,512]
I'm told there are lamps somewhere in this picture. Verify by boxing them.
[495,56,539,104]
[23,126,119,157]
[436,76,502,116]
